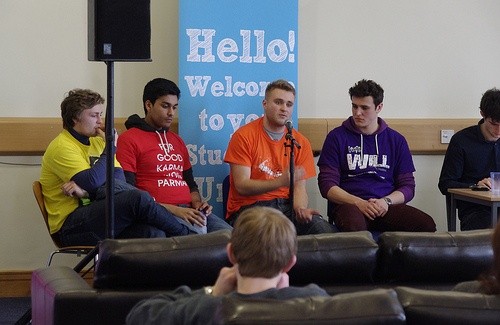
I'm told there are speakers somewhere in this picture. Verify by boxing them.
[88,0,153,62]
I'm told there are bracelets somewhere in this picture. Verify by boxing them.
[469,183,476,189]
[208,289,212,293]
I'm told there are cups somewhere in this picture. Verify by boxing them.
[490,172,500,196]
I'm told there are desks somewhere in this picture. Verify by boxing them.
[447,188,500,232]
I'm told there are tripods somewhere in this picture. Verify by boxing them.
[13,63,114,325]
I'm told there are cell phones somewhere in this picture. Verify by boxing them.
[470,185,490,191]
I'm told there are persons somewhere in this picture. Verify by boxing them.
[438,89,500,231]
[40,88,198,248]
[223,80,337,235]
[127,207,330,325]
[117,78,234,235]
[317,80,436,233]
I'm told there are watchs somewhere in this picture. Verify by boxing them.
[384,196,392,205]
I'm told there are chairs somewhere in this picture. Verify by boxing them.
[32,180,102,278]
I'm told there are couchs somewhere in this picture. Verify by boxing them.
[30,228,500,325]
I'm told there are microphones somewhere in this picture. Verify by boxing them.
[285,119,293,137]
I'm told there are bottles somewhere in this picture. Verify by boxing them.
[193,209,207,234]
